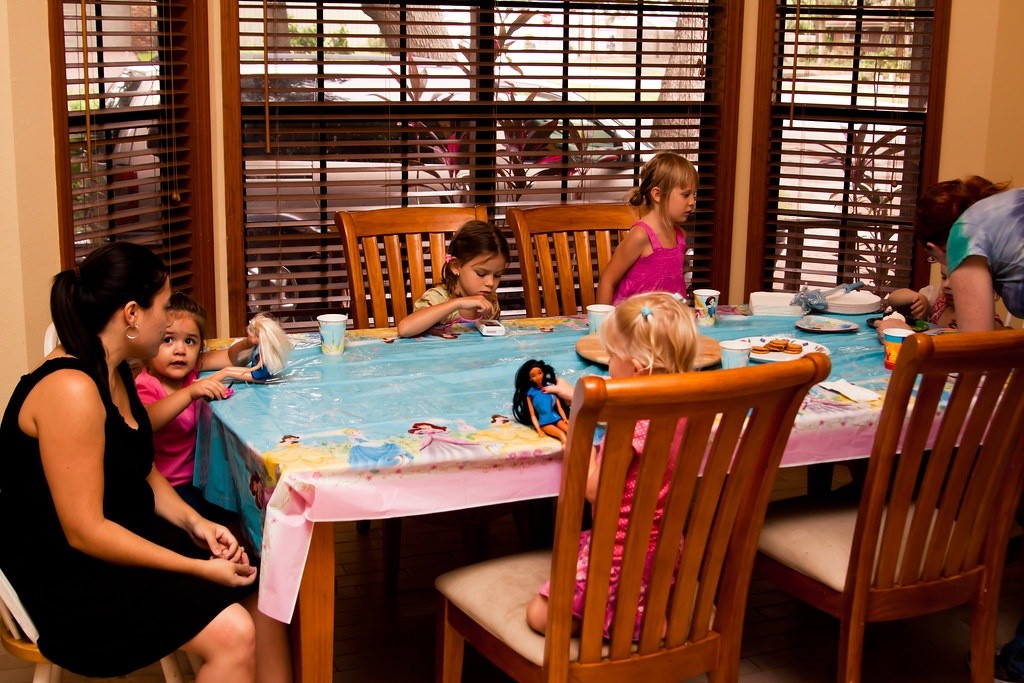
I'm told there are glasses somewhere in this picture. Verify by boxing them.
[927,255,947,264]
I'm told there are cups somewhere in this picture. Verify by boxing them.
[883,328,916,370]
[719,341,753,369]
[586,304,614,335]
[316,314,348,356]
[693,289,721,328]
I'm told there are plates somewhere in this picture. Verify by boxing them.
[795,319,860,334]
[866,318,929,333]
[822,287,882,314]
[734,336,830,363]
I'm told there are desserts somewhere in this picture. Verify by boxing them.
[882,305,916,325]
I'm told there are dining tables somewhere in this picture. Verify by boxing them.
[193,305,1023,683]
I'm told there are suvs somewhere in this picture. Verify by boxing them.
[88,55,786,324]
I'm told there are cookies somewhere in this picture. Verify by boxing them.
[752,340,803,354]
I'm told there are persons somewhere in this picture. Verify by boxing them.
[135,292,259,525]
[527,293,700,640]
[193,312,291,385]
[874,176,1024,346]
[888,262,1004,331]
[512,359,570,446]
[595,153,699,308]
[0,242,294,683]
[397,219,511,337]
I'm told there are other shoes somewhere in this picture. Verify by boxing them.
[967,637,1024,683]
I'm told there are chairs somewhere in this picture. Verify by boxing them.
[334,204,489,328]
[0,568,205,683]
[432,352,832,683]
[507,203,641,318]
[750,328,1023,682]
